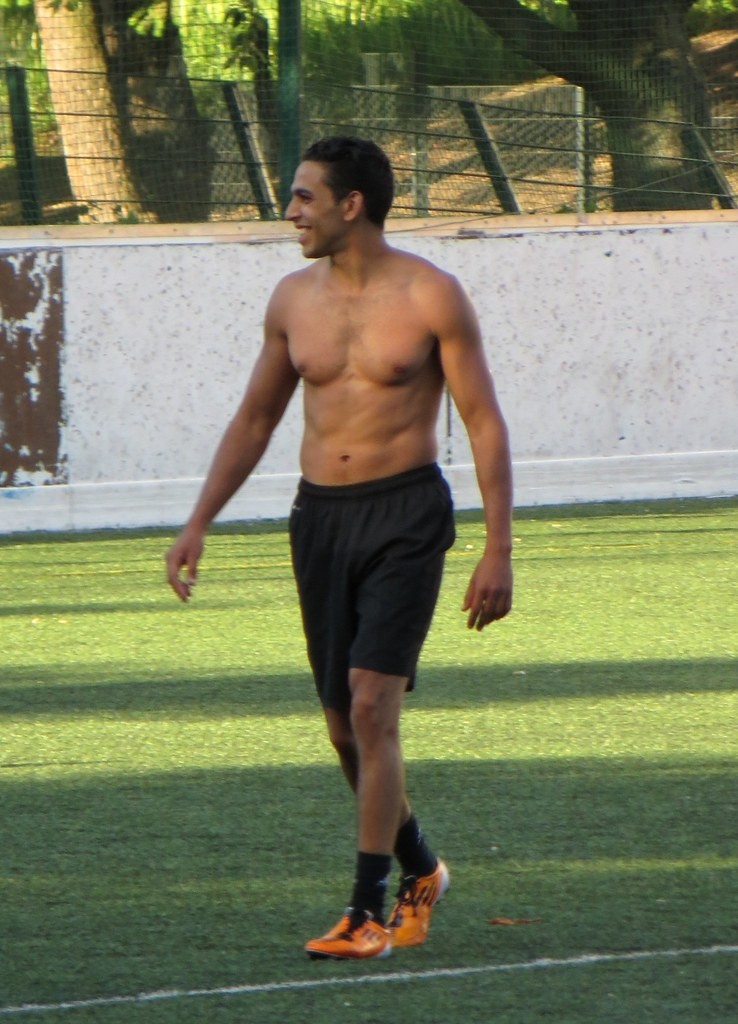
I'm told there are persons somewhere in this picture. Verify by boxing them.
[165,135,512,961]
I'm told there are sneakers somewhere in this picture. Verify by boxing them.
[305,909,396,964]
[391,858,451,945]
